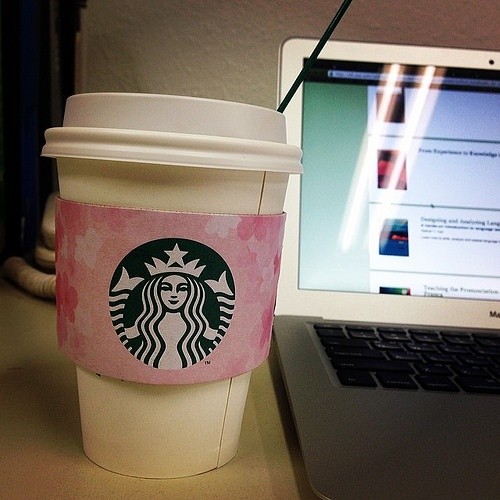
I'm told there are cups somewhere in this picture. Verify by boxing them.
[40,91,305,481]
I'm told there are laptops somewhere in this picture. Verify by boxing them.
[271,36,499,500]
